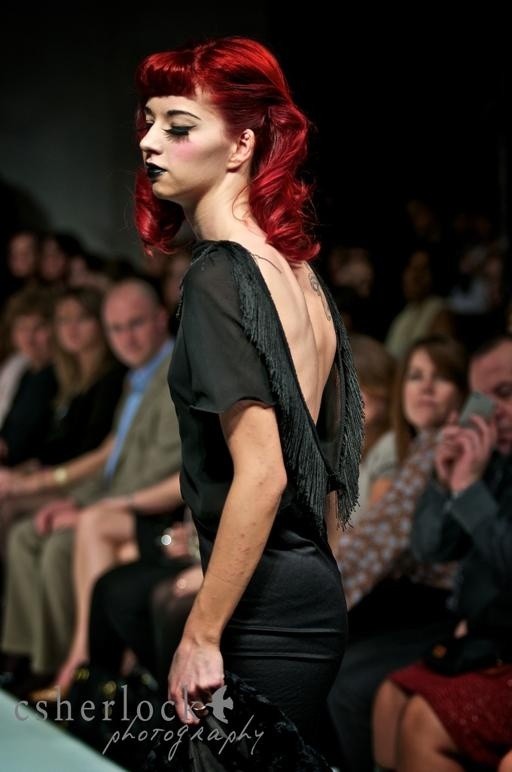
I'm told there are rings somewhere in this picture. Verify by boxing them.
[193,704,208,711]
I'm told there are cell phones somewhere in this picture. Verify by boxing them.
[457,390,496,430]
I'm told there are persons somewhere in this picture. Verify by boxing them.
[129,37,368,769]
[0,200,509,771]
